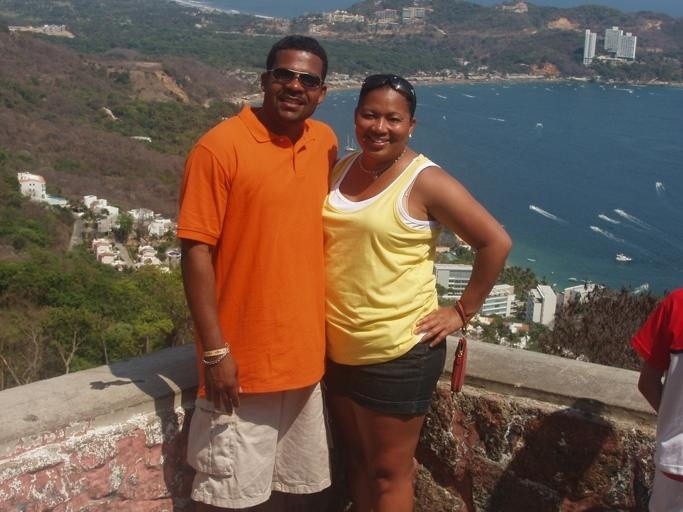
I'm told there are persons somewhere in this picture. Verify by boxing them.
[173,36,340,512]
[322,71,511,509]
[627,289,683,511]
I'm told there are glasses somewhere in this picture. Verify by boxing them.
[359,73,416,111]
[267,67,325,88]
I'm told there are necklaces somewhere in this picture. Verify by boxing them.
[356,145,409,177]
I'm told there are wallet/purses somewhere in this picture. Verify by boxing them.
[450,299,468,393]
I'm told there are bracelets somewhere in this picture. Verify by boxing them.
[203,347,228,367]
[455,299,476,330]
